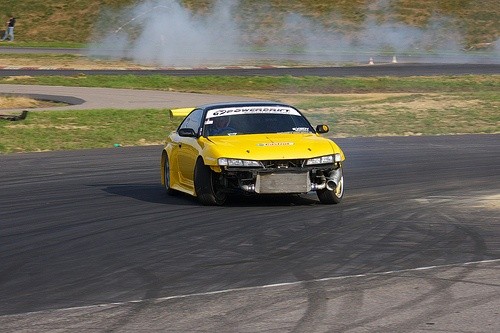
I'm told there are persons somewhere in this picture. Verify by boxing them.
[2,15,16,41]
[209,115,232,134]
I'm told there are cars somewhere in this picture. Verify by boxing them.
[159,101,346,207]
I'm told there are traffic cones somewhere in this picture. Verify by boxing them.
[391,55,397,63]
[369,58,373,64]
